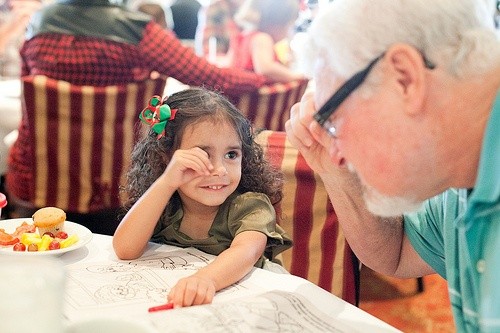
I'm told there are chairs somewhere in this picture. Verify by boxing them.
[22,75,166,215]
[249,131,363,308]
[223,80,308,132]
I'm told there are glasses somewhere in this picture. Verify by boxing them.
[312,49,435,138]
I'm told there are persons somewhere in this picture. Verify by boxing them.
[0,0,287,218]
[136,0,328,85]
[285,0,500,333]
[113,88,293,309]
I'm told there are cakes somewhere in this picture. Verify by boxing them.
[33,206,66,237]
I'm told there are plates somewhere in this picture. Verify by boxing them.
[0,218,94,258]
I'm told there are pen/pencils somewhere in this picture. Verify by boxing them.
[147,304,175,312]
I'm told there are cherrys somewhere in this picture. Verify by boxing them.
[43,232,54,238]
[13,243,25,251]
[48,241,60,250]
[55,232,68,239]
[28,243,38,251]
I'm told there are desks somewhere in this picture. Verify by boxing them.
[0,233,405,333]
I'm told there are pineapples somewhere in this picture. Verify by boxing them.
[22,233,78,251]
